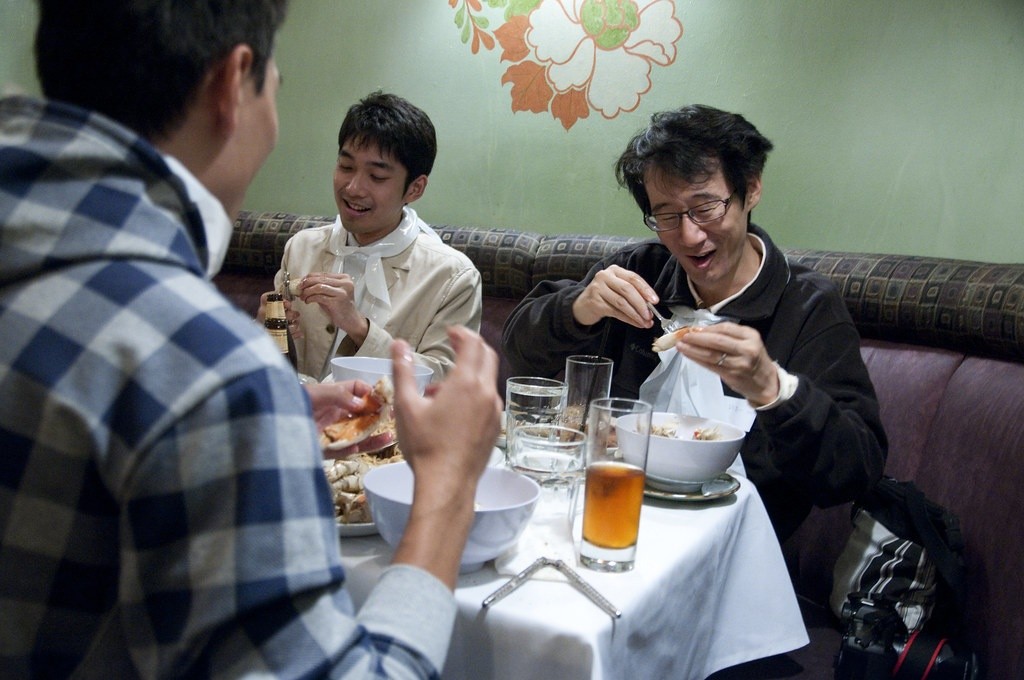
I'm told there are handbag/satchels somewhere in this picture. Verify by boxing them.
[828,475,986,656]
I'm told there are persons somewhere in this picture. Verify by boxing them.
[255,93,483,383]
[0,0,501,679]
[502,104,889,546]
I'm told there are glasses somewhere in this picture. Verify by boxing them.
[643,187,739,232]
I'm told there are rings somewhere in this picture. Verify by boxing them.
[717,353,726,366]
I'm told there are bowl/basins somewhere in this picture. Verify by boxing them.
[615,413,746,494]
[330,355,434,401]
[363,460,541,576]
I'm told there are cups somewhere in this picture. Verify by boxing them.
[580,398,653,571]
[511,425,586,537]
[560,355,613,453]
[505,376,567,468]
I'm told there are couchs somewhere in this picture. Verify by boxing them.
[211,209,1024,680]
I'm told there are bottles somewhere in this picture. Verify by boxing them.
[265,293,298,374]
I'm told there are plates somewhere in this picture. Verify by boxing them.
[495,410,507,447]
[334,521,379,536]
[643,474,740,500]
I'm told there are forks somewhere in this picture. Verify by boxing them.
[647,302,677,334]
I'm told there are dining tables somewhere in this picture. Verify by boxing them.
[334,410,812,680]
